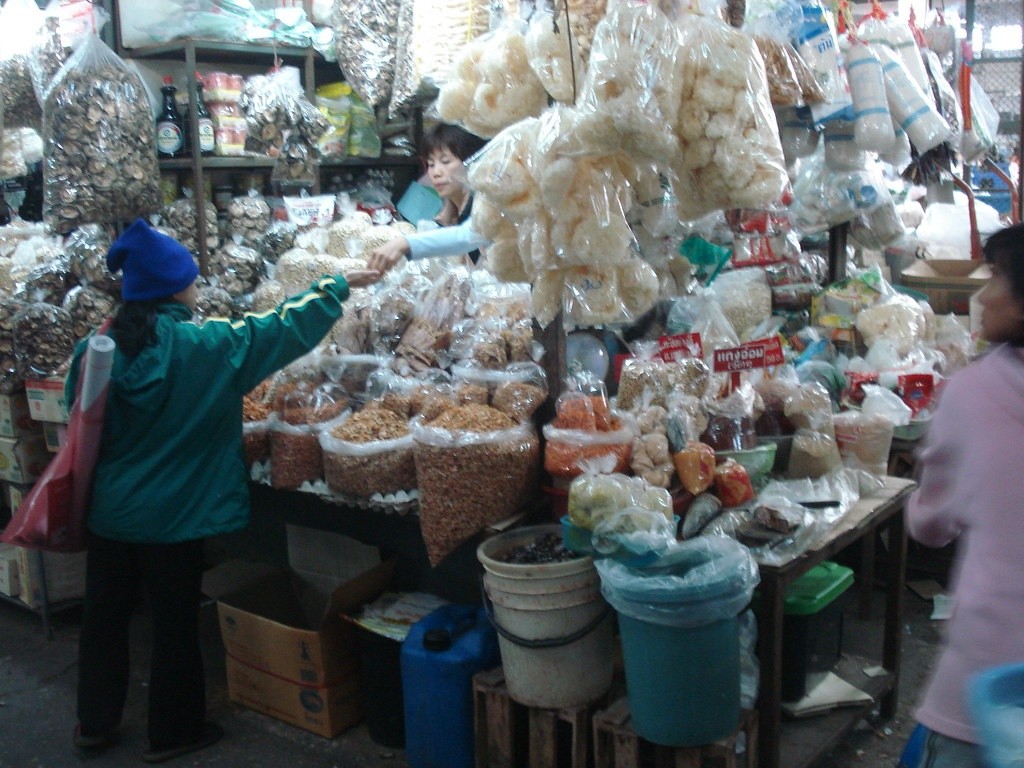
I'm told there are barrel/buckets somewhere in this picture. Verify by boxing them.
[399,599,500,767]
[595,535,741,746]
[475,523,613,709]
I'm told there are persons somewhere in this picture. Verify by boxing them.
[364,121,491,274]
[65,218,380,760]
[897,223,1024,768]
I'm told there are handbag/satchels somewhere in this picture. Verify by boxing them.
[1,317,112,554]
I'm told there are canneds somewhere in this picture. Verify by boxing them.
[212,185,235,240]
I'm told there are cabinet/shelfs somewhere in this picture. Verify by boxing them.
[110,0,422,282]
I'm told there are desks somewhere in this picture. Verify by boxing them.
[754,476,918,767]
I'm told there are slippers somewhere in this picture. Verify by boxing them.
[142,721,222,763]
[73,722,121,748]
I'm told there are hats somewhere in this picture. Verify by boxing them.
[106,219,199,304]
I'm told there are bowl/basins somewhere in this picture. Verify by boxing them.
[759,436,794,458]
[711,441,778,475]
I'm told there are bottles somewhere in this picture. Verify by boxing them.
[155,74,184,159]
[183,70,215,156]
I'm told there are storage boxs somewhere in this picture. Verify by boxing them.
[0,378,89,608]
[198,523,396,739]
